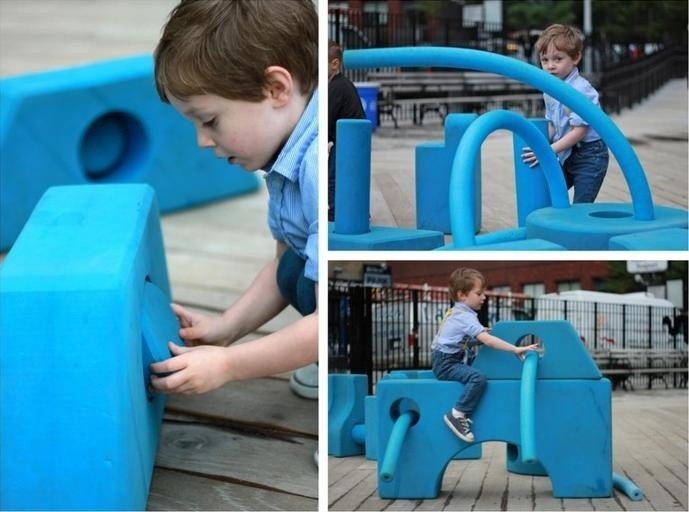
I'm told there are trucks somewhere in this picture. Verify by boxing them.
[334,294,533,369]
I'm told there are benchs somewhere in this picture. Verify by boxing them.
[587,348,689,392]
[372,68,546,126]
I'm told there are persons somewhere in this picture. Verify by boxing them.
[328,43,367,221]
[430,268,538,443]
[521,24,608,204]
[146,0,319,400]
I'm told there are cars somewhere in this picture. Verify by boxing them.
[481,27,665,73]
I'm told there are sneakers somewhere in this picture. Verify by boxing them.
[443,407,475,444]
[289,362,318,400]
